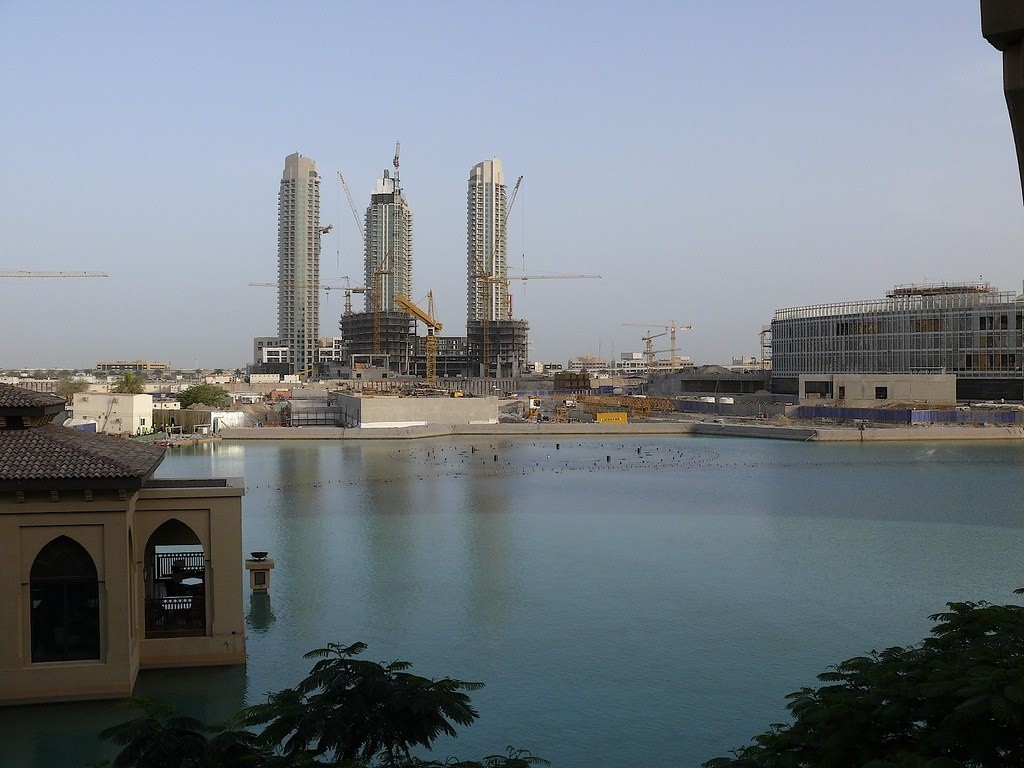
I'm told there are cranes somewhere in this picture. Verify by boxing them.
[0,270,110,278]
[621,320,694,368]
[641,330,667,369]
[337,171,394,354]
[248,277,372,316]
[393,142,401,310]
[470,174,601,377]
[392,290,443,381]
[643,347,682,367]
